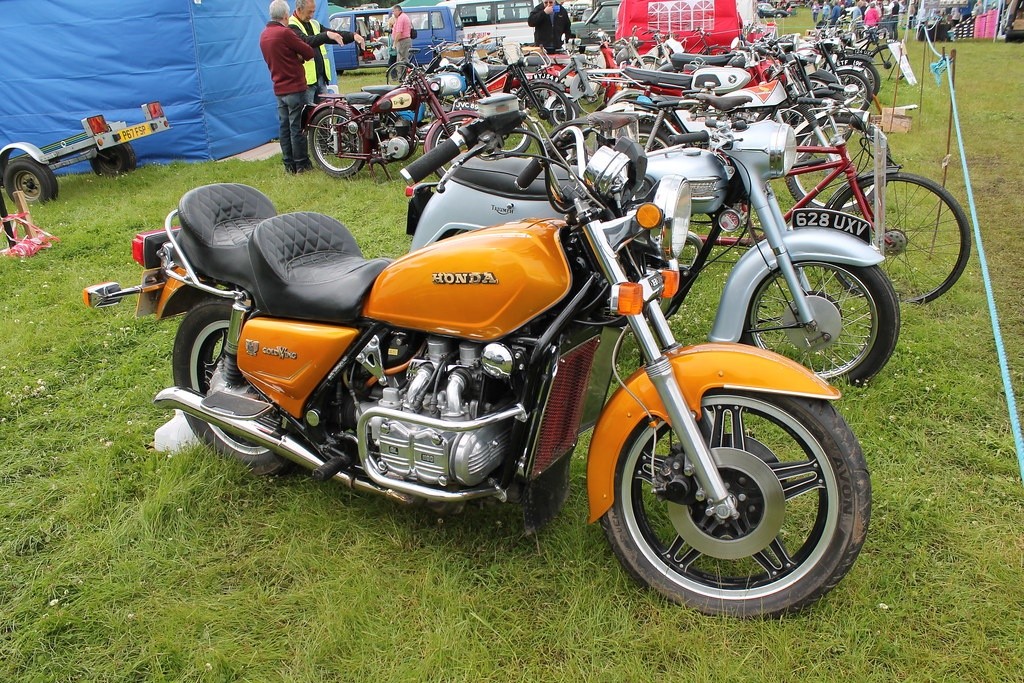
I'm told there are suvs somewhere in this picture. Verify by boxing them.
[569,1,622,45]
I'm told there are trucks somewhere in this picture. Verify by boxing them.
[571,3,590,22]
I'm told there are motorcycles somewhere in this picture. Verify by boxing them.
[299,20,972,395]
[78,90,874,624]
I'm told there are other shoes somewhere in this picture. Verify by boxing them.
[282,158,297,173]
[294,158,314,173]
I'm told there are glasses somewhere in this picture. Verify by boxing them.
[544,2,554,4]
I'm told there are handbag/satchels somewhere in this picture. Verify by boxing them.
[410,22,417,39]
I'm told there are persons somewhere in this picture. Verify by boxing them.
[777,0,792,14]
[387,5,414,80]
[289,0,366,154]
[796,0,906,43]
[908,0,1011,35]
[528,0,571,53]
[374,21,382,36]
[260,0,316,173]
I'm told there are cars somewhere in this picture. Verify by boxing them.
[757,3,790,19]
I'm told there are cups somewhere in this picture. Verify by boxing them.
[553,5,560,12]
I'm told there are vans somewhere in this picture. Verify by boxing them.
[427,0,536,46]
[328,6,456,75]
